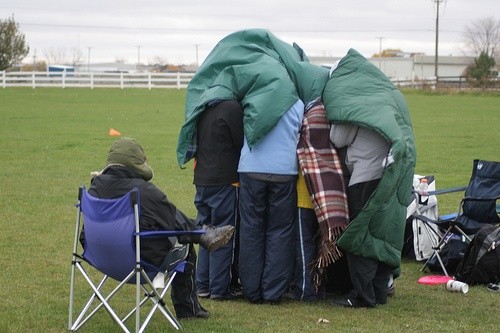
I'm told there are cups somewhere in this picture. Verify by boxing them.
[446,280,469,293]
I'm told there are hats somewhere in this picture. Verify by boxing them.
[104,138,154,180]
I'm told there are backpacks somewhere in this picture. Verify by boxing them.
[456,224,500,291]
[428,238,471,276]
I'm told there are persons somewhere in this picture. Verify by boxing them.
[79,137,237,319]
[180,30,417,308]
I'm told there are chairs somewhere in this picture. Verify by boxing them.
[68,187,206,333]
[411,159,500,277]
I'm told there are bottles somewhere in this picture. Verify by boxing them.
[417,179,429,215]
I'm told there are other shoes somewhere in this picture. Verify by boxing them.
[210,285,238,300]
[199,222,235,250]
[334,295,362,307]
[196,288,210,299]
[177,300,210,320]
